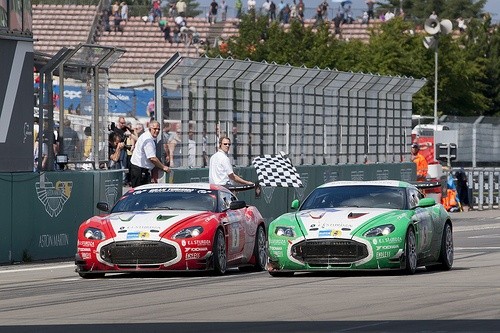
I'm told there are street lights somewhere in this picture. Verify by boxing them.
[472,115,485,168]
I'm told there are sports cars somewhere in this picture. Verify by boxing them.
[74,182,267,279]
[264,179,454,277]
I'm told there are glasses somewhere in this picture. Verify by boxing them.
[152,128,160,130]
[222,143,230,145]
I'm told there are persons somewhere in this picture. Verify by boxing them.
[129,121,171,189]
[87,0,500,59]
[210,136,255,185]
[455,167,475,211]
[33,87,237,173]
[411,143,428,197]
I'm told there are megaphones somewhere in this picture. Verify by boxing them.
[422,36,436,49]
[424,18,440,35]
[440,19,452,34]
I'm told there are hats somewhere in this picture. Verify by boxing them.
[411,143,418,148]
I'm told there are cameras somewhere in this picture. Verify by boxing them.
[124,145,132,151]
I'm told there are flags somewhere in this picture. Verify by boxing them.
[252,151,304,188]
